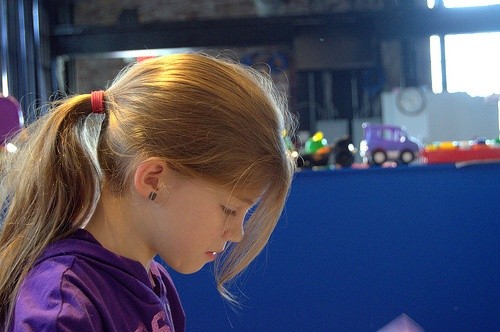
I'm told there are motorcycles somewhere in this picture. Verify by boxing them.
[302,131,355,168]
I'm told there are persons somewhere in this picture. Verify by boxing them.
[0,53,291,332]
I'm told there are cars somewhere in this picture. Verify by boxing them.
[361,123,419,166]
